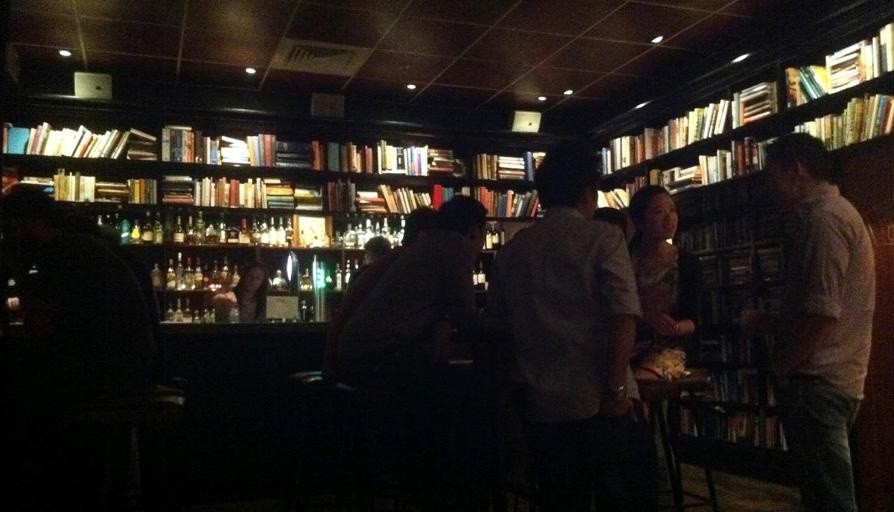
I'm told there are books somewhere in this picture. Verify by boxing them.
[593,21,894,451]
[2,166,543,217]
[2,122,545,181]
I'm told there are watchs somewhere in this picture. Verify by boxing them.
[607,380,625,398]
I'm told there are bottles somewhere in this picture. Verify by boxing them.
[149,250,359,294]
[94,208,505,247]
[162,294,315,324]
[471,257,487,291]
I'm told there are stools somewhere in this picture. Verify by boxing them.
[639,377,719,512]
[77,385,185,512]
[280,370,410,512]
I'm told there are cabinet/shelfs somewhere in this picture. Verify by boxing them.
[0,91,565,324]
[580,0,894,489]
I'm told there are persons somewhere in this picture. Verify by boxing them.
[740,135,876,510]
[326,184,706,510]
[210,258,270,321]
[0,184,156,511]
[507,145,666,508]
